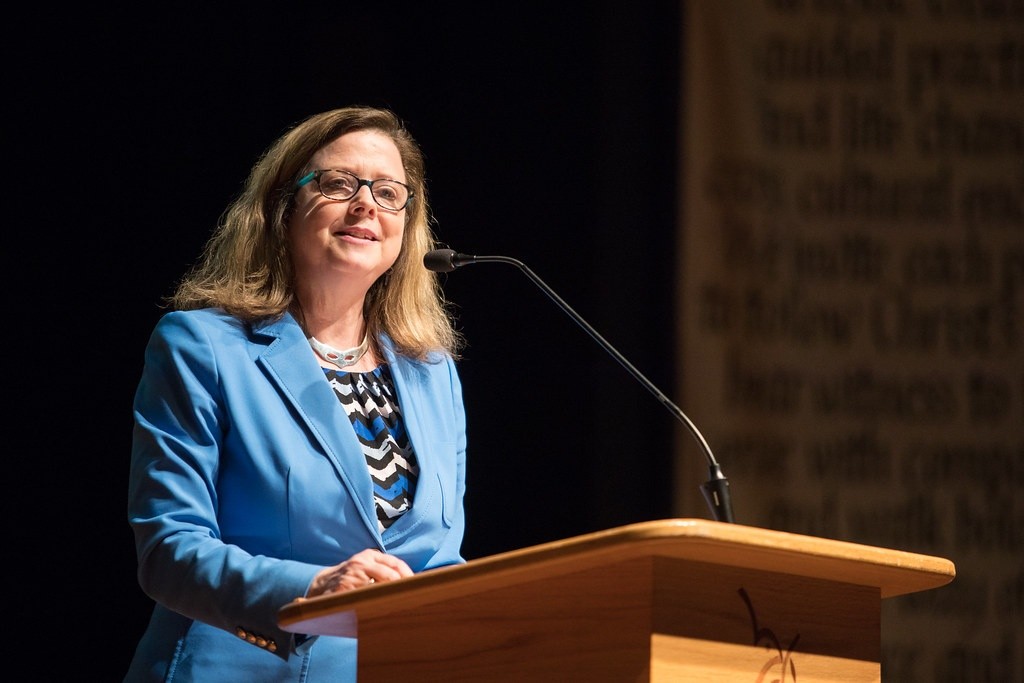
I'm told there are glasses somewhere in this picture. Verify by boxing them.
[292,169,415,212]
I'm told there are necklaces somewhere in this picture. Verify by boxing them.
[300,323,370,369]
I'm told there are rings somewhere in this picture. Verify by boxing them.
[365,578,375,585]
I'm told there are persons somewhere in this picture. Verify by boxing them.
[123,106,473,683]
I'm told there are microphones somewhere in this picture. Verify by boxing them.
[423,249,730,522]
